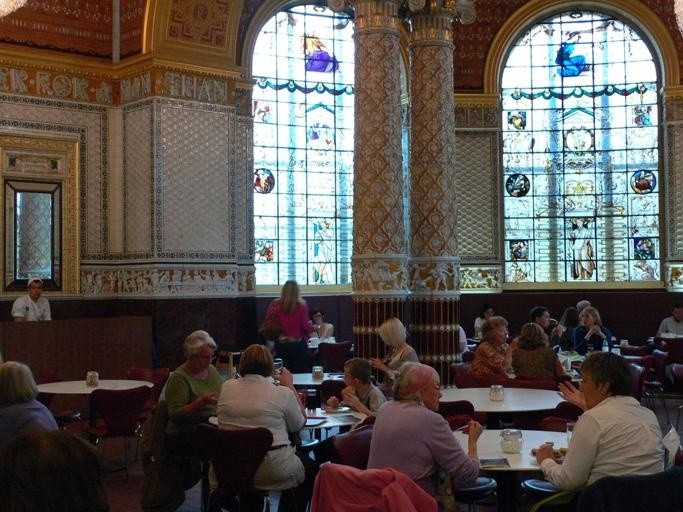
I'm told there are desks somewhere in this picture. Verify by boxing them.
[37,380,155,472]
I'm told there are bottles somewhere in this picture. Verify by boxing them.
[489,385,504,401]
[307,388,317,417]
[602,336,630,357]
[500,429,522,454]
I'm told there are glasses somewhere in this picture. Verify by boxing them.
[199,354,217,360]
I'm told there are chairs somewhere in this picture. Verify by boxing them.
[34,372,60,408]
[89,337,683,512]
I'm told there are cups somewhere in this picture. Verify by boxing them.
[273,358,285,386]
[566,424,573,443]
[328,338,335,344]
[313,366,324,381]
[310,337,319,346]
[87,371,99,386]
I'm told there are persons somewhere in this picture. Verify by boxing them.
[364,361,485,504]
[262,280,316,371]
[367,316,419,386]
[11,277,52,322]
[458,296,616,379]
[1,429,111,512]
[313,357,388,462]
[656,300,683,384]
[1,361,59,430]
[209,344,313,512]
[310,305,335,338]
[165,330,237,512]
[530,351,665,512]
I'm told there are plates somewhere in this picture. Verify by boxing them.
[328,406,349,413]
